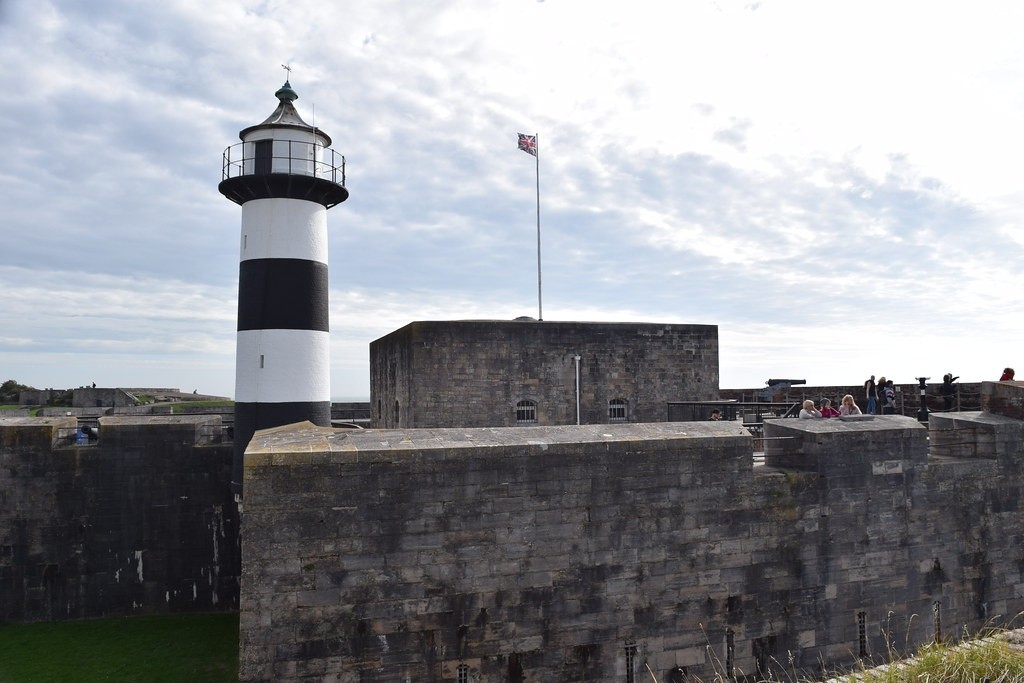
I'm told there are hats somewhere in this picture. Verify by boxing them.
[820,398,828,407]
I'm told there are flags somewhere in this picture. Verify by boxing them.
[517,132,536,157]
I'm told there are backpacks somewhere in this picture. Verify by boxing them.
[880,389,889,405]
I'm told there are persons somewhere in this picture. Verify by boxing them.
[882,380,896,415]
[839,395,862,415]
[819,398,840,418]
[707,409,720,421]
[863,376,879,415]
[878,377,887,415]
[940,374,959,412]
[1000,368,1014,381]
[799,400,822,419]
